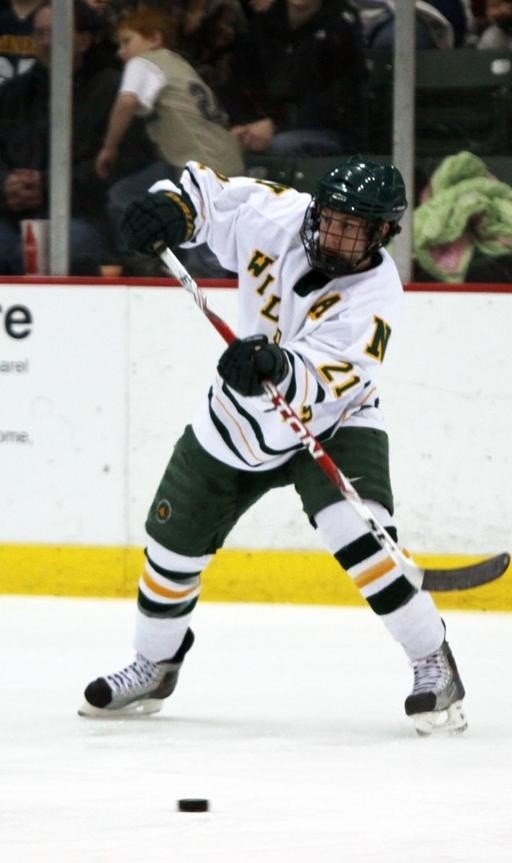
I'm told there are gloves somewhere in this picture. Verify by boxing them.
[217,334,289,396]
[119,189,197,257]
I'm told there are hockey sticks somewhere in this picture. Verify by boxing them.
[158,246,511,592]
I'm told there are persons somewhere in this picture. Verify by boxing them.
[84,144,463,714]
[1,0,512,285]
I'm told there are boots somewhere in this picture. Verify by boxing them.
[405,641,465,716]
[85,653,183,710]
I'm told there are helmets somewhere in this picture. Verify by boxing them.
[300,155,407,273]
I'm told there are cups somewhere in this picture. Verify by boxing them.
[20,221,44,273]
